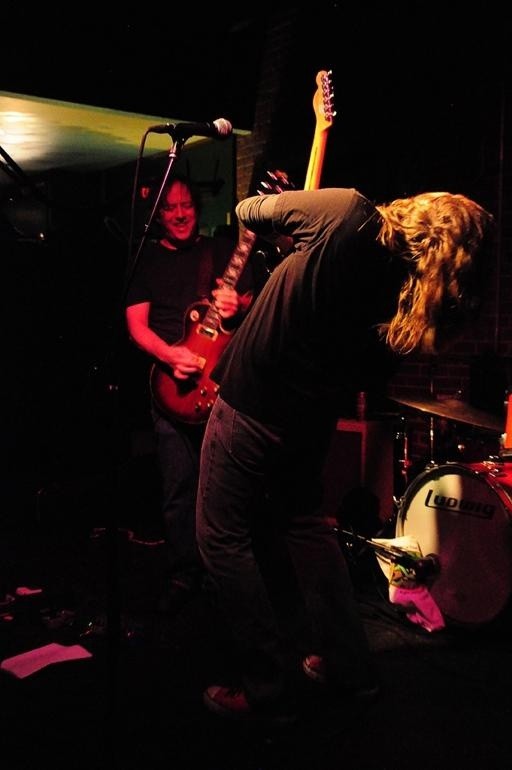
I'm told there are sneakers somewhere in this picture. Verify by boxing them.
[301,654,379,697]
[203,684,297,725]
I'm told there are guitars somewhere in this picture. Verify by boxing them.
[149,169,295,426]
[243,71,337,317]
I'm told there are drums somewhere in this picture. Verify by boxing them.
[396,459,512,627]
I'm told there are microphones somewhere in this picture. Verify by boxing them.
[150,115,233,141]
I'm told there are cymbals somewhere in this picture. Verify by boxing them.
[393,397,506,432]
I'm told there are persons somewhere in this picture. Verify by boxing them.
[124,177,252,382]
[182,178,499,728]
